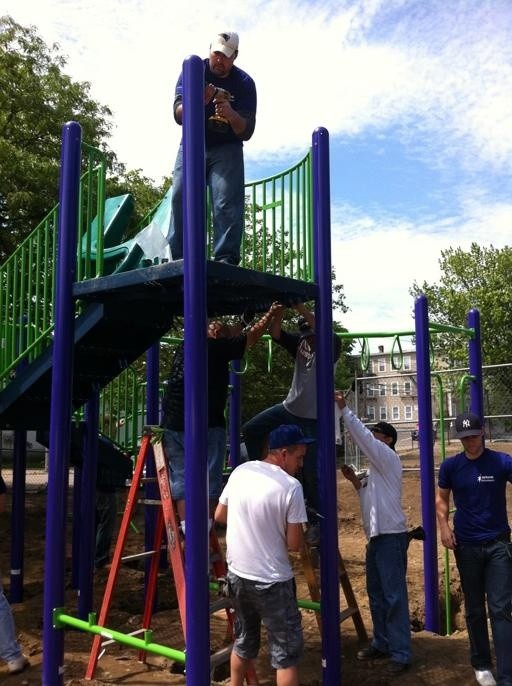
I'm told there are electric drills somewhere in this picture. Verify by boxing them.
[205,81,235,134]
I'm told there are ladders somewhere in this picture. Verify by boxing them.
[85,425,259,686]
[224,436,368,645]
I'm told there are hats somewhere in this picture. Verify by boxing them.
[375,422,397,451]
[297,314,315,338]
[269,423,316,449]
[210,32,238,57]
[454,414,481,439]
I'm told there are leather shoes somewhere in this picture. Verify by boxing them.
[357,648,387,660]
[382,662,410,675]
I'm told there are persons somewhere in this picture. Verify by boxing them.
[165,32,257,267]
[93,415,132,568]
[434,412,512,686]
[240,301,340,545]
[213,424,317,686]
[335,389,413,674]
[0,476,30,672]
[159,300,287,539]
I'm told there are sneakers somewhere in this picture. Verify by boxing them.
[474,668,496,686]
[8,655,29,673]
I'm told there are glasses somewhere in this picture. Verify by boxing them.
[370,428,383,434]
